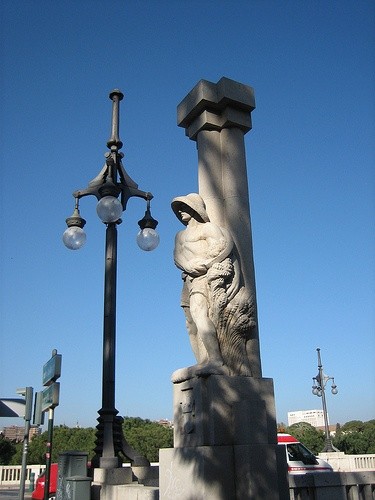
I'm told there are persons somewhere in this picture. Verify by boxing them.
[170,192,230,377]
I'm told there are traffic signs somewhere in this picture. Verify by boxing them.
[41,382,59,411]
[42,353,60,385]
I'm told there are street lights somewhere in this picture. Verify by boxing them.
[312,347,338,452]
[62,89,160,486]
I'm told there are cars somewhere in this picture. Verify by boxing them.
[31,463,90,500]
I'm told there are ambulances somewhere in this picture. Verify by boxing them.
[277,431,333,476]
